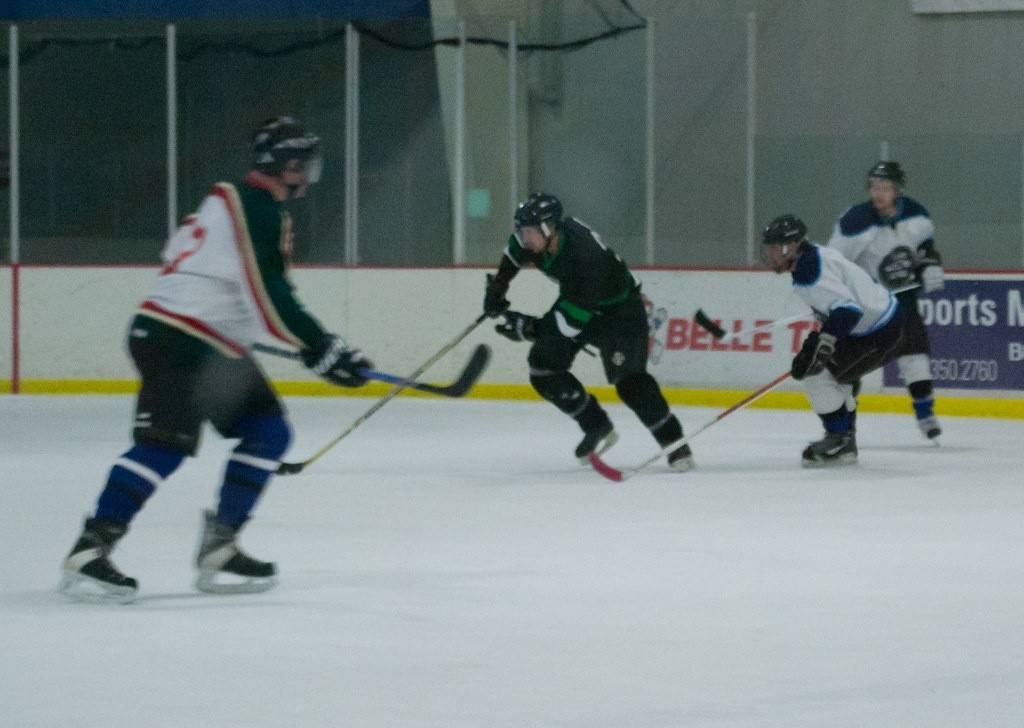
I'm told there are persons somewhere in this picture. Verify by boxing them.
[824,160,949,449]
[483,194,697,473]
[56,113,375,606]
[757,215,910,469]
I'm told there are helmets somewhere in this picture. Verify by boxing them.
[868,161,904,184]
[762,215,807,243]
[251,115,320,175]
[514,191,562,225]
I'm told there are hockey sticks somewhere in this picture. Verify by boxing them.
[253,342,489,397]
[588,370,795,483]
[275,311,490,477]
[693,282,924,342]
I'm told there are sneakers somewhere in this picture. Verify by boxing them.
[802,428,858,469]
[574,394,620,465]
[59,516,139,606]
[918,415,942,447]
[196,510,281,593]
[651,413,695,472]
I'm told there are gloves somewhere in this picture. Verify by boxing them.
[495,311,541,343]
[791,331,833,380]
[919,261,946,293]
[301,334,375,392]
[483,273,510,319]
